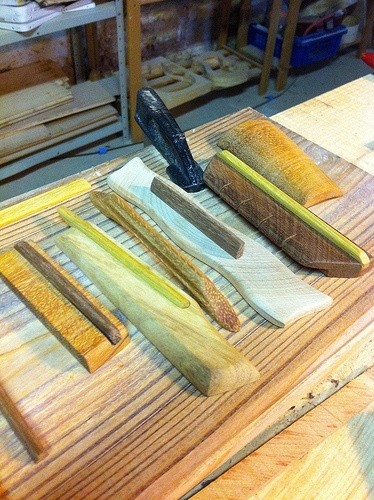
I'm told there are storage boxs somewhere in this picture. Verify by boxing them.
[246,20,350,69]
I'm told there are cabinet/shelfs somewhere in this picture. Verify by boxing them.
[0,0,129,183]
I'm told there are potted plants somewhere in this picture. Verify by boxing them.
[339,15,359,46]
